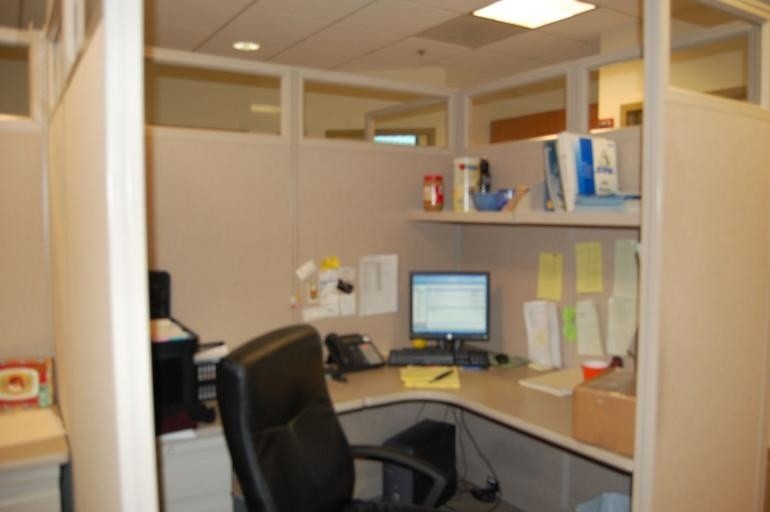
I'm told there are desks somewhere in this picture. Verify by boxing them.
[157,336,635,512]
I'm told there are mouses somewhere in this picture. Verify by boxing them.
[496,354,508,364]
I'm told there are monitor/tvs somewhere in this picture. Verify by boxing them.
[409,270,490,351]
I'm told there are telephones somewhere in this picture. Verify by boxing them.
[324,333,386,373]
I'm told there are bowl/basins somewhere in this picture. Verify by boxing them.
[472,192,501,210]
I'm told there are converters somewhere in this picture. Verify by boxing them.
[472,488,495,502]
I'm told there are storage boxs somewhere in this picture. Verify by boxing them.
[570,357,635,457]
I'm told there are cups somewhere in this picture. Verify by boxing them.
[581,360,608,381]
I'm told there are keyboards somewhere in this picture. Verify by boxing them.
[387,347,488,368]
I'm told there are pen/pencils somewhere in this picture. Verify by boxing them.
[429,369,454,383]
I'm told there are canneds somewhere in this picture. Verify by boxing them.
[423,175,444,211]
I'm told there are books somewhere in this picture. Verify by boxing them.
[518,367,582,398]
[538,129,641,216]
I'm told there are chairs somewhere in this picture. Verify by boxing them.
[214,321,453,511]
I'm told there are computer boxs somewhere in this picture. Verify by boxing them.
[382,418,457,508]
